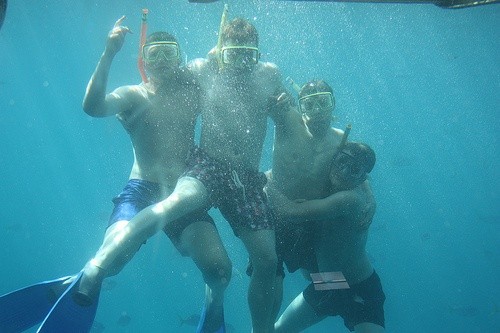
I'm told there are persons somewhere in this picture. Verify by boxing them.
[37,4,298,333]
[245,74,350,333]
[263,122,385,333]
[0,8,232,333]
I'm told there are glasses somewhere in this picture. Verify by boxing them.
[332,149,370,177]
[143,40,180,62]
[298,91,334,115]
[221,45,260,68]
